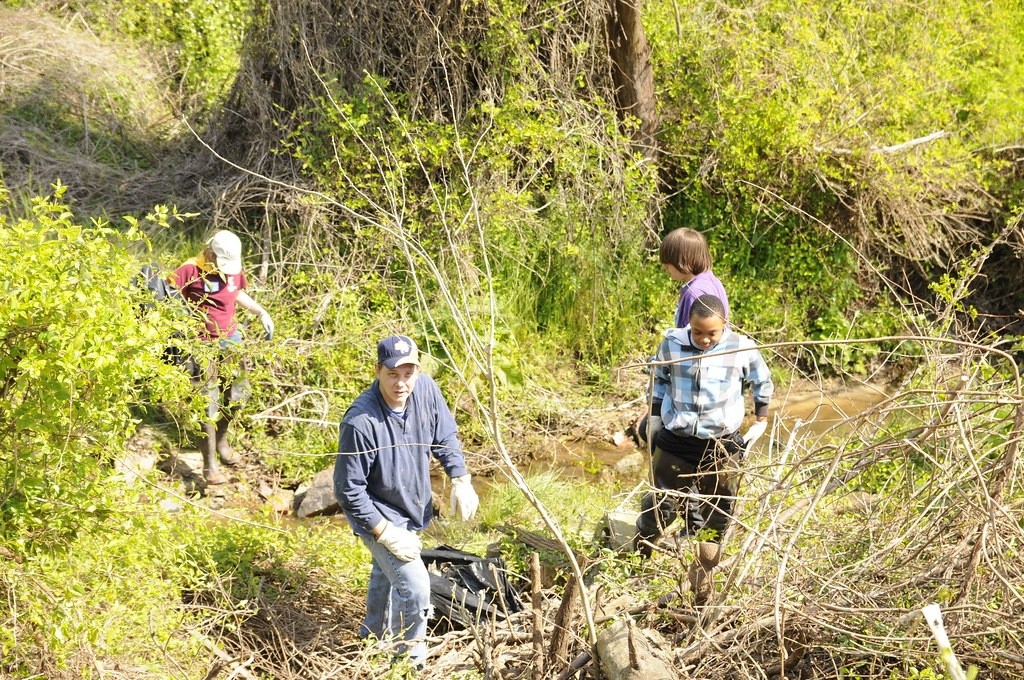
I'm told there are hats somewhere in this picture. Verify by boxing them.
[376,334,422,369]
[212,230,242,275]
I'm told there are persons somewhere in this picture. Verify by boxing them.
[334,336,479,671]
[631,227,774,597]
[166,230,274,484]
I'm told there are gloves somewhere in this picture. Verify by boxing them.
[644,416,664,455]
[448,473,479,521]
[258,312,276,341]
[740,420,768,461]
[376,521,423,561]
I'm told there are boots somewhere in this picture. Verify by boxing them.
[214,403,243,465]
[626,527,657,577]
[198,420,230,485]
